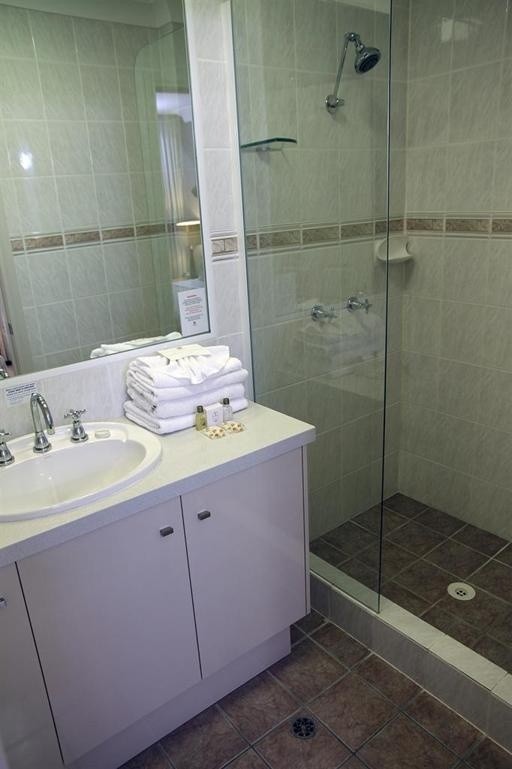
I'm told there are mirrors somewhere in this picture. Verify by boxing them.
[0,0,210,383]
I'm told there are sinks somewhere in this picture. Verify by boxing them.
[0,422,164,523]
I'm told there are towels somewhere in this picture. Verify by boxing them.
[121,345,249,435]
[91,331,181,359]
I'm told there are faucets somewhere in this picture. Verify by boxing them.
[0,369,9,378]
[31,392,57,450]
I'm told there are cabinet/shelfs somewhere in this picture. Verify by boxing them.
[0,562,64,769]
[15,447,307,767]
